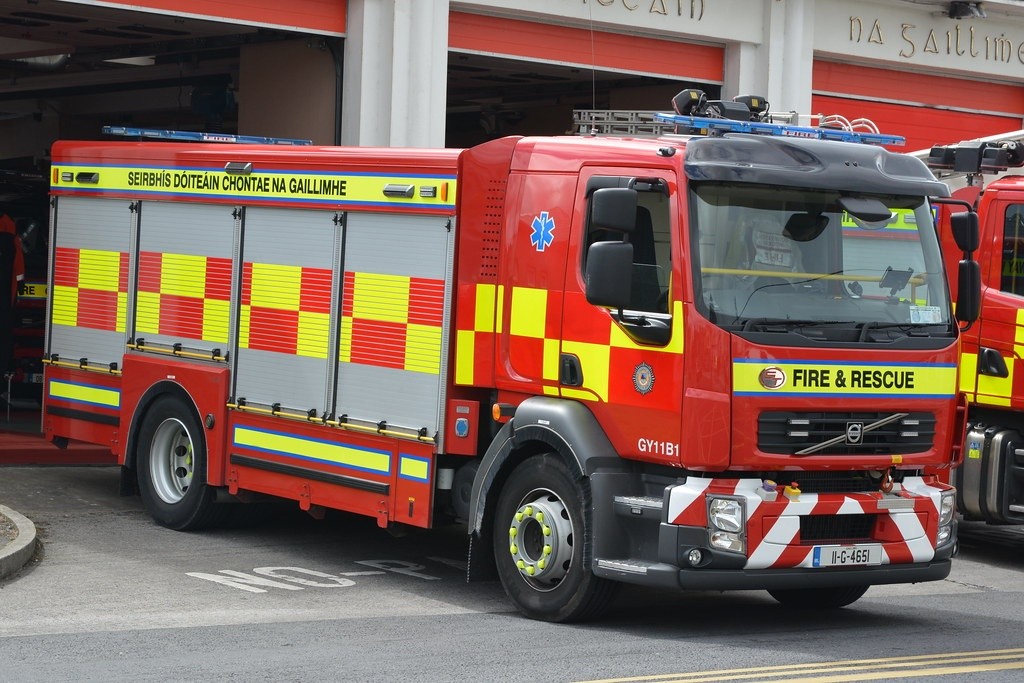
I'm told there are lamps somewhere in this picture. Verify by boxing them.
[949,1,987,20]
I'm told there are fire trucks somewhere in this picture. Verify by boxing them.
[839,174,1024,552]
[0,192,49,421]
[39,88,979,627]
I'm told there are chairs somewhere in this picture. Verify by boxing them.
[592,207,669,312]
[723,210,813,289]
